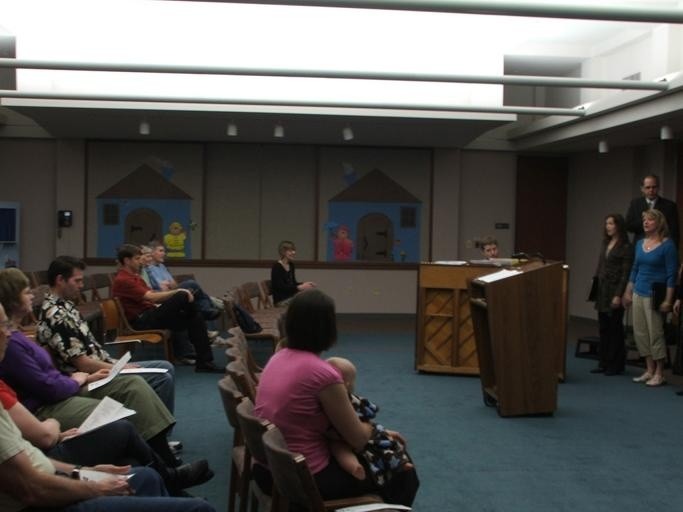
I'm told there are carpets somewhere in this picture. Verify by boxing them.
[168,314,683,512]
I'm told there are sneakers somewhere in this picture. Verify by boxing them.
[210,296,223,303]
[216,302,224,308]
[168,440,183,453]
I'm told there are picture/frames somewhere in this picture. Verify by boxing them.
[204,140,314,269]
[83,137,209,267]
[313,142,435,269]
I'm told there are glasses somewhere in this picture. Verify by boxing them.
[23,289,34,296]
[1,321,12,328]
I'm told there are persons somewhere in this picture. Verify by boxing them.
[674,265,683,394]
[110,244,226,374]
[145,240,224,321]
[325,357,374,480]
[0,403,217,511]
[138,244,226,346]
[0,302,218,499]
[586,212,635,376]
[270,240,316,307]
[1,267,185,468]
[34,255,184,453]
[481,237,500,259]
[623,208,678,387]
[625,174,677,244]
[256,288,420,512]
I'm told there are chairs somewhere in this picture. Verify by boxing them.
[90,273,111,304]
[241,281,288,314]
[218,374,255,511]
[114,296,172,360]
[100,299,162,344]
[224,294,278,329]
[260,280,288,308]
[76,275,103,322]
[227,287,278,322]
[236,397,384,510]
[262,424,398,511]
[225,347,259,389]
[226,359,262,405]
[226,338,247,371]
[234,287,283,320]
[34,270,47,286]
[227,326,263,378]
[223,297,280,352]
[24,272,36,289]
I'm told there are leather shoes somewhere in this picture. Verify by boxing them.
[607,369,624,375]
[676,387,682,397]
[199,470,214,484]
[646,375,666,386]
[207,330,218,338]
[203,309,220,320]
[195,361,226,374]
[172,459,210,489]
[590,367,605,372]
[633,372,650,382]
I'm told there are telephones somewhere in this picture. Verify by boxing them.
[56,209,73,240]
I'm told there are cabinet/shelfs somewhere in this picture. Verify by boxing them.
[466,257,564,419]
[414,264,569,380]
